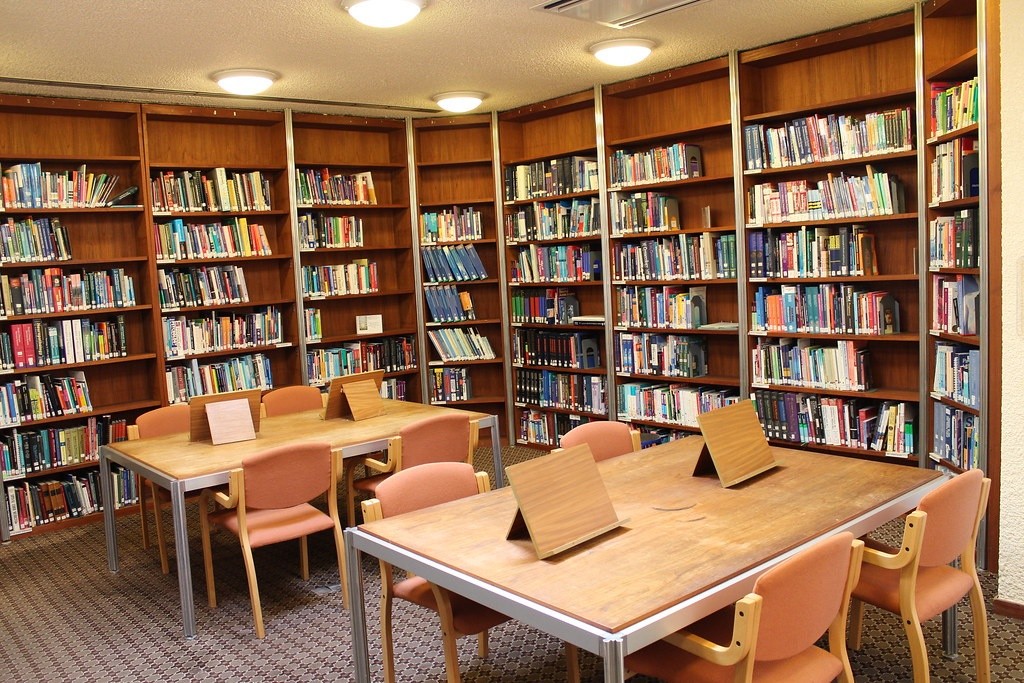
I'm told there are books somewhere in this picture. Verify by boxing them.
[421,206,494,404]
[746,108,915,456]
[297,168,418,403]
[0,162,138,533]
[503,156,611,444]
[609,143,739,451]
[929,76,981,565]
[149,167,280,406]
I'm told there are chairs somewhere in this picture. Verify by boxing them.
[848,467,992,682]
[199,440,351,637]
[622,530,864,683]
[360,460,512,683]
[550,420,641,466]
[261,385,324,418]
[346,414,479,529]
[135,403,202,576]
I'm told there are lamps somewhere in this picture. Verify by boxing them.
[208,68,281,97]
[431,91,487,113]
[588,38,655,68]
[340,0,428,29]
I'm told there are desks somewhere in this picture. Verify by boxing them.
[342,435,955,683]
[98,394,504,636]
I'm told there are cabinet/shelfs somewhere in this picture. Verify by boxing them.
[0,0,1010,577]
